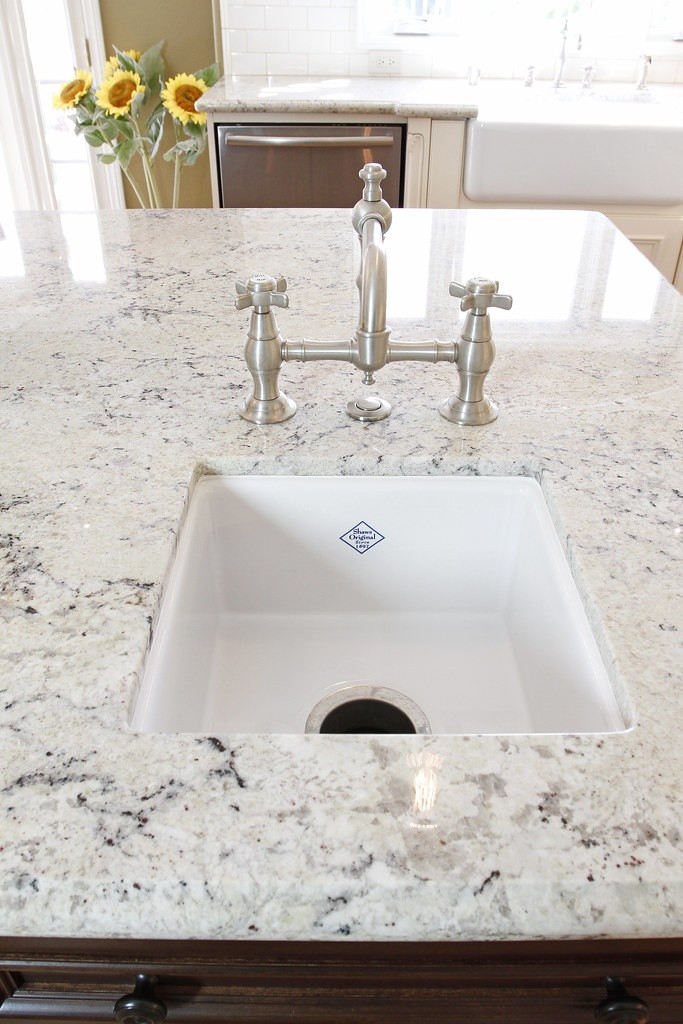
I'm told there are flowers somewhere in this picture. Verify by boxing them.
[56,39,221,209]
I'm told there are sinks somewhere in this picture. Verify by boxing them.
[465,90,683,205]
[130,456,635,737]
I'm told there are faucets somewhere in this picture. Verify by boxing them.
[350,162,395,384]
[551,18,569,87]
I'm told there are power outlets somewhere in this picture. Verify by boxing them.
[368,49,403,74]
